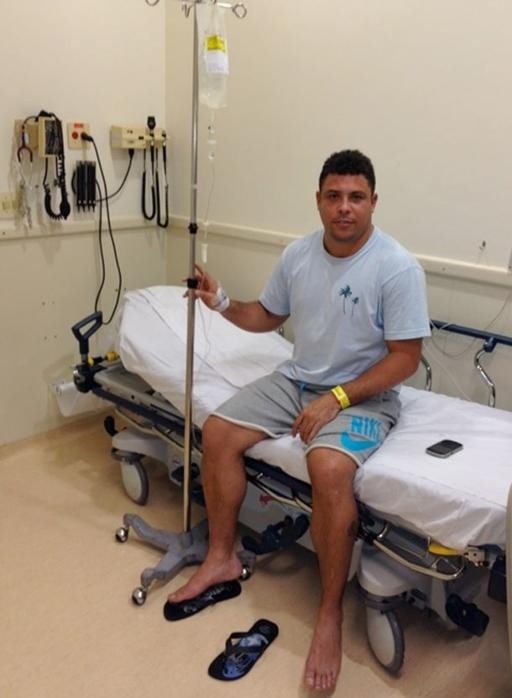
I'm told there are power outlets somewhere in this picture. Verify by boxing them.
[67,122,90,149]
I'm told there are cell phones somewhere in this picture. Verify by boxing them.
[426,439,463,460]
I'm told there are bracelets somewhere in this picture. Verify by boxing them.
[330,384,351,411]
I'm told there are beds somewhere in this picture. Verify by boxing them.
[70,285,512,674]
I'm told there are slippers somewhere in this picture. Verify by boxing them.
[164,581,249,621]
[208,619,279,681]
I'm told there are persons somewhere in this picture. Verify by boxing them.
[166,148,433,694]
